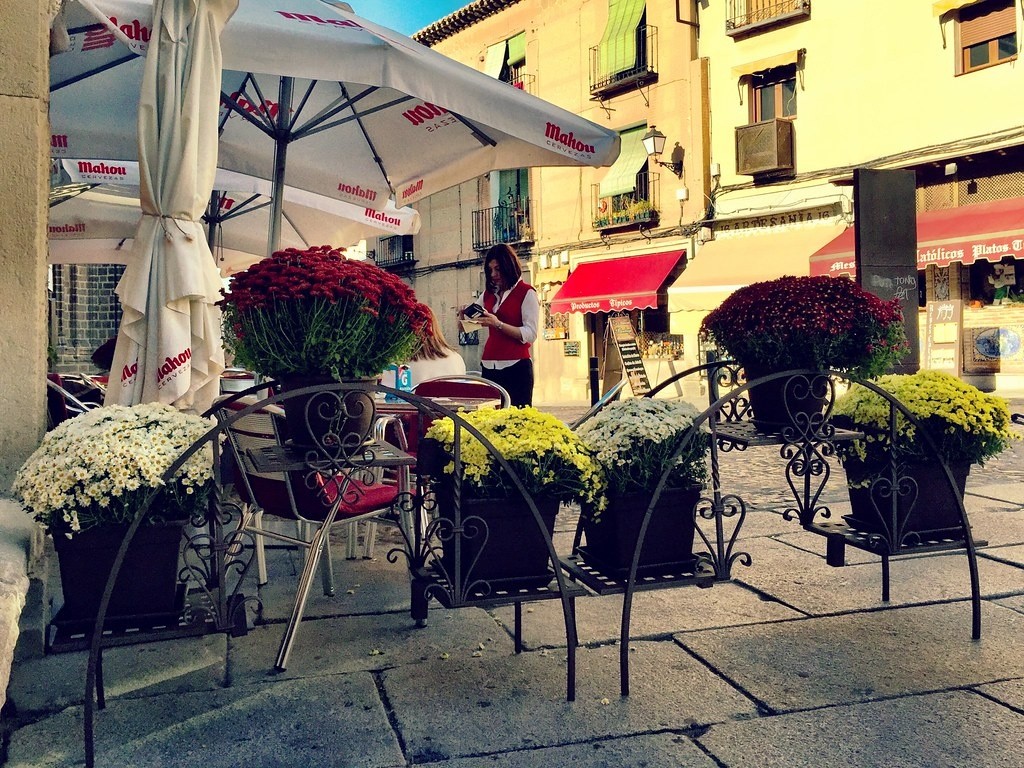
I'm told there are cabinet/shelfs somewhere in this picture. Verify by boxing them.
[43,375,590,768]
[560,355,990,696]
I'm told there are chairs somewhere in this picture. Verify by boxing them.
[212,368,509,673]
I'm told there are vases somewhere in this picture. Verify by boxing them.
[742,356,827,434]
[268,369,378,446]
[428,483,562,581]
[578,475,702,567]
[833,415,972,540]
[44,519,193,615]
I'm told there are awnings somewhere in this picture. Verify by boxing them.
[550,249,686,314]
[809,196,1024,278]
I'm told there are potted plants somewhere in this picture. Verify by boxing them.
[598,201,657,227]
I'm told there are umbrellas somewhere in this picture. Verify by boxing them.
[49,158,423,259]
[104,0,241,424]
[46,238,259,276]
[49,0,621,259]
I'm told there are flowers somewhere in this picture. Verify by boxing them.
[11,401,228,538]
[214,244,432,382]
[572,395,721,498]
[423,402,609,513]
[831,370,1022,490]
[699,274,912,382]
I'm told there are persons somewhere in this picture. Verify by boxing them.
[988,264,1007,305]
[457,244,540,409]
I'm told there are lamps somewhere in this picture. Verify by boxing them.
[641,125,682,179]
[945,162,959,178]
